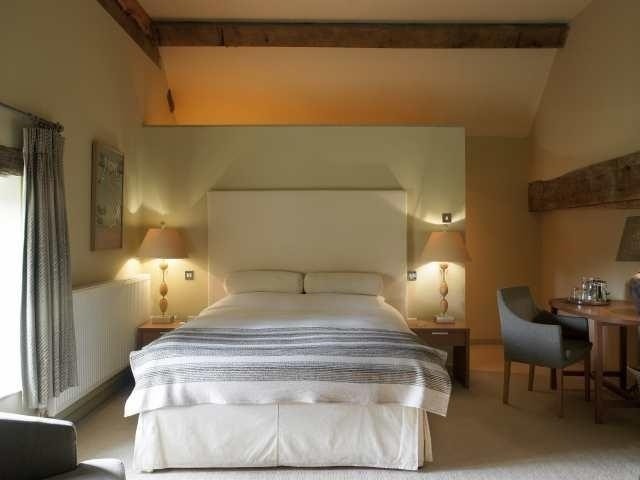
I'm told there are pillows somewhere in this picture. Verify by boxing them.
[224,270,304,294]
[304,271,383,295]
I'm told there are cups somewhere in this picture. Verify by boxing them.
[569,274,611,305]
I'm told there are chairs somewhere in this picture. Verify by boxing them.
[497,285,592,418]
[0,412,125,480]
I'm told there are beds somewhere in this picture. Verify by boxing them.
[123,189,452,472]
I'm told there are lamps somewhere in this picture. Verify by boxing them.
[139,220,187,324]
[422,224,472,323]
[615,216,640,262]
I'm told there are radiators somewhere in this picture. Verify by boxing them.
[48,275,151,418]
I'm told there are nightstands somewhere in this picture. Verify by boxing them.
[136,320,182,349]
[409,320,470,388]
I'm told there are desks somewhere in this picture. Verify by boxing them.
[548,297,640,424]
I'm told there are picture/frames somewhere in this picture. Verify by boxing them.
[91,137,124,251]
